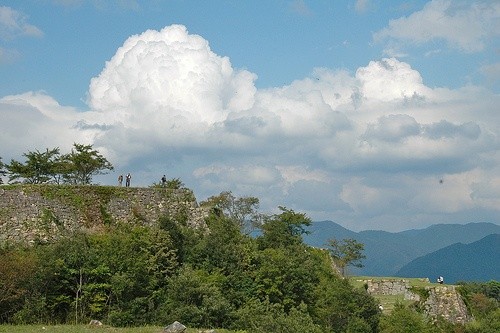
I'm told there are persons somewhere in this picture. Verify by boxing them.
[162,175,166,186]
[118,176,123,186]
[379,305,383,310]
[437,276,443,284]
[126,173,131,187]
